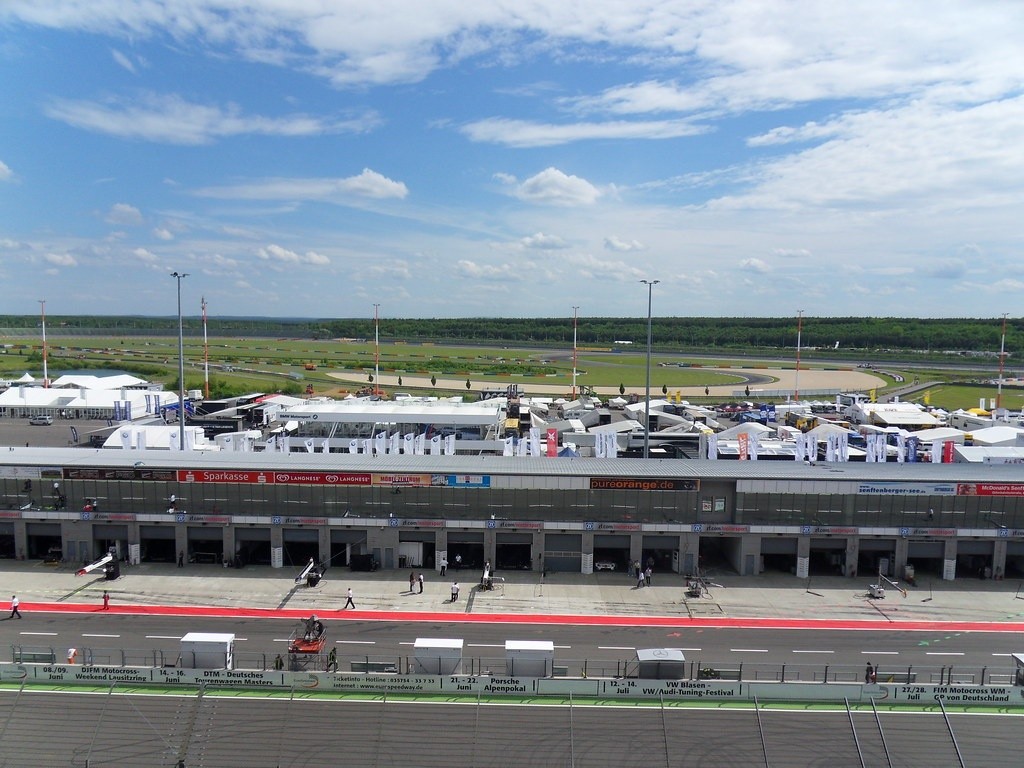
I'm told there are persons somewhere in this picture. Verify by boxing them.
[647,557,654,573]
[328,646,339,671]
[9,596,22,619]
[276,654,284,669]
[67,647,78,664]
[451,582,459,602]
[410,572,417,594]
[419,573,424,594]
[93,500,97,510]
[344,588,355,609]
[637,571,645,587]
[456,554,461,571]
[54,482,60,495]
[439,557,449,576]
[177,550,184,568]
[646,567,652,586]
[635,560,640,578]
[103,590,110,610]
[930,508,934,521]
[170,493,175,507]
[865,661,873,683]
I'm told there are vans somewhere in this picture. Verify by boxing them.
[29,416,53,425]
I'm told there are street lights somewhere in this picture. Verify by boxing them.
[372,303,381,396]
[36,300,50,389]
[199,295,210,399]
[571,306,581,402]
[996,311,1011,409]
[169,272,191,450]
[793,308,807,401]
[637,279,663,459]
